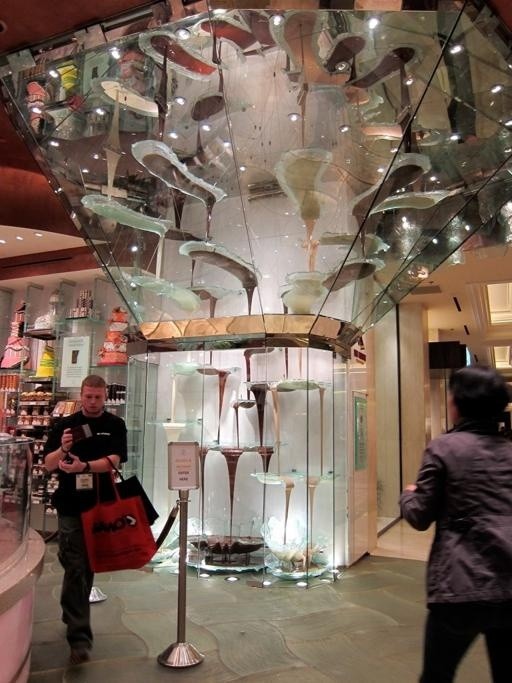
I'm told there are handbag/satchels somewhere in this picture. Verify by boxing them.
[80,456,159,573]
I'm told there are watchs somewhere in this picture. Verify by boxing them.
[82,461,91,473]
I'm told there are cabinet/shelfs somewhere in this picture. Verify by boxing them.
[0,316,147,534]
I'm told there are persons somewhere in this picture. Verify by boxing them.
[398,362,511,683]
[40,373,158,668]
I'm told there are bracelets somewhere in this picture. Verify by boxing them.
[61,445,69,453]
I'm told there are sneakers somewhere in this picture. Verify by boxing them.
[69,641,91,664]
